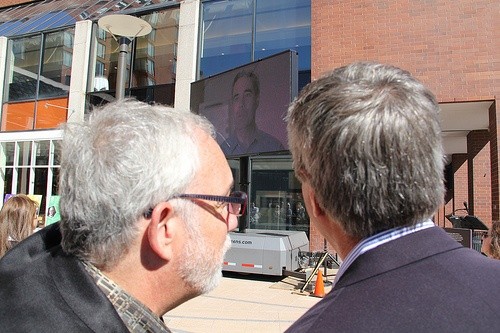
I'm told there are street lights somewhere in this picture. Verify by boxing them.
[98,15,152,102]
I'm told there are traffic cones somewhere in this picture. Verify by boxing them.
[308,269,325,297]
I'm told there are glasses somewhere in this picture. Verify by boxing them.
[481,233,495,240]
[143,190,249,220]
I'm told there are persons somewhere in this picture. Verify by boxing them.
[0,98,247,333]
[0,195,38,260]
[246,201,306,223]
[278,59,499,333]
[219,71,284,156]
[49,206,55,216]
